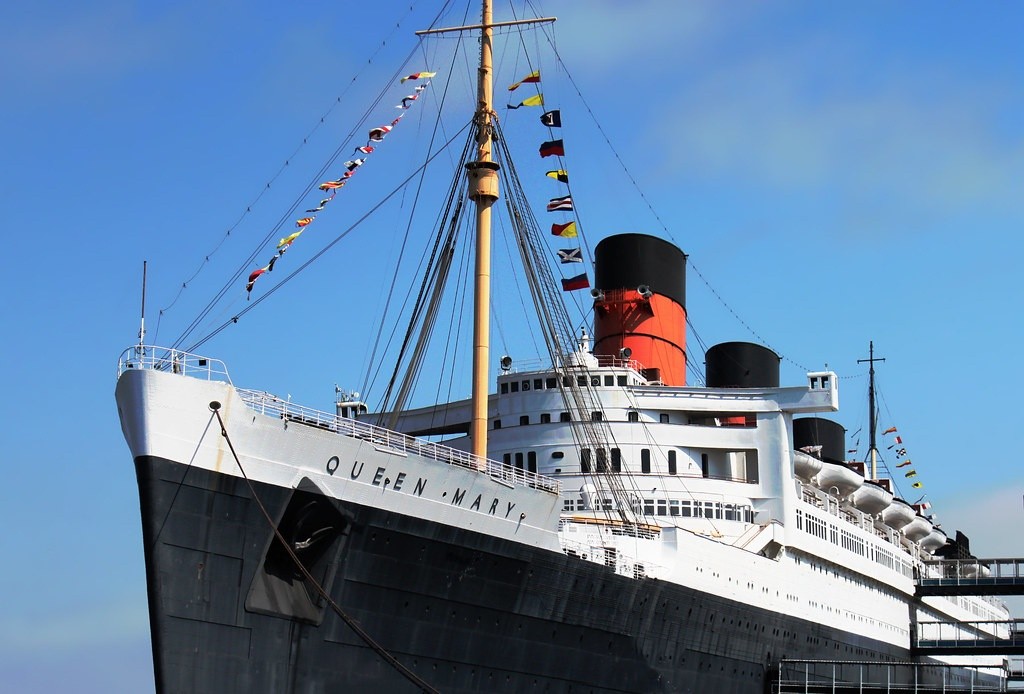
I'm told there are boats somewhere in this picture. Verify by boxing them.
[116,0,1024,693]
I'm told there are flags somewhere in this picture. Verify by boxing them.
[507,92,544,109]
[561,273,590,291]
[558,247,584,264]
[547,194,573,212]
[247,71,436,289]
[540,109,562,128]
[881,427,938,520]
[546,170,567,183]
[551,221,578,238]
[508,72,541,91]
[540,139,565,158]
